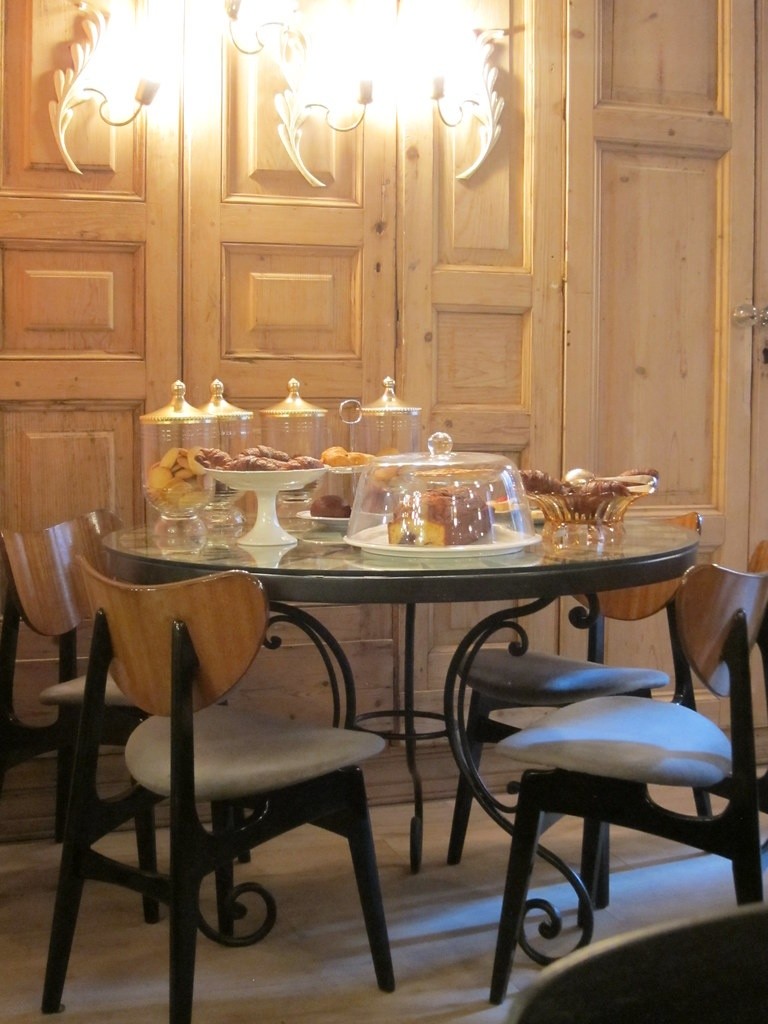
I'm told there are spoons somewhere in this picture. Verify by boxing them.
[565,469,656,485]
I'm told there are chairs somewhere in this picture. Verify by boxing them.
[486,542,768,1006]
[0,506,240,924]
[40,554,394,1024]
[447,512,722,911]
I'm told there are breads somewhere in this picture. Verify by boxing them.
[200,444,325,471]
[517,469,658,497]
[386,485,495,547]
[320,445,399,466]
[310,494,351,518]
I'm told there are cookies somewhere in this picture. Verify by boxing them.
[147,446,213,512]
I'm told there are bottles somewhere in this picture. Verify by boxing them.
[259,378,328,517]
[200,380,254,528]
[350,376,422,498]
[140,381,217,556]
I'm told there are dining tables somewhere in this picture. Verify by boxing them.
[86,506,720,941]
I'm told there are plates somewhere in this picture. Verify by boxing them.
[203,464,329,546]
[360,510,393,519]
[344,523,542,558]
[487,500,510,515]
[297,511,350,530]
[531,510,545,524]
[521,469,660,542]
